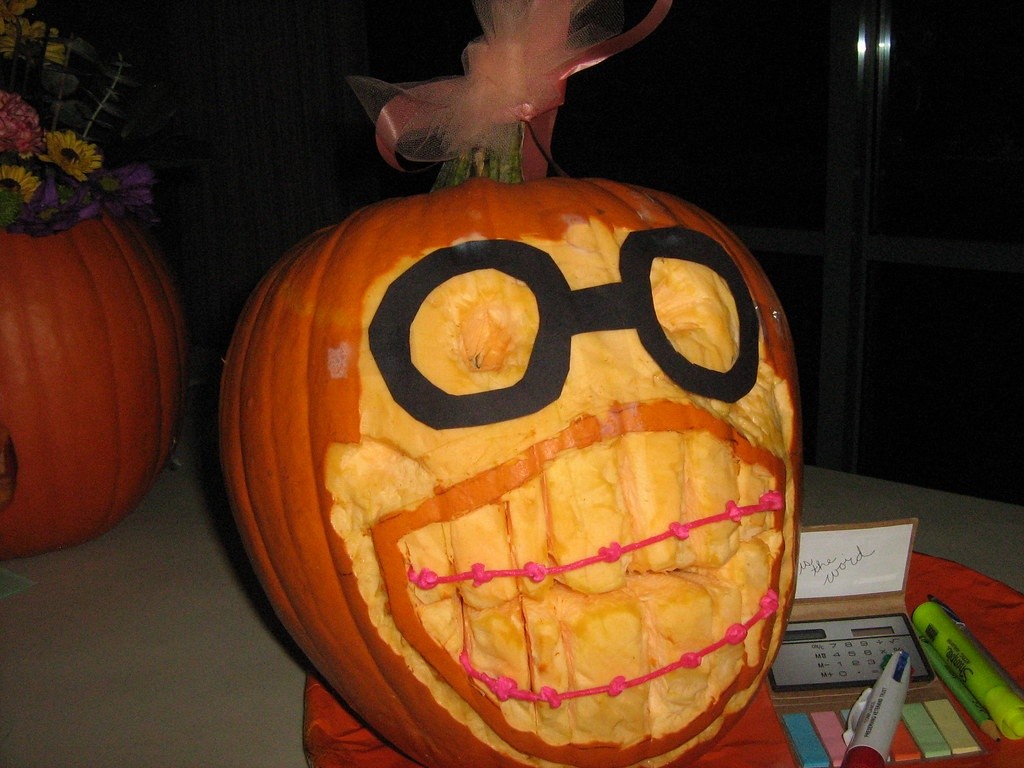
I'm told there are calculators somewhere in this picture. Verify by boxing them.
[767,613,935,692]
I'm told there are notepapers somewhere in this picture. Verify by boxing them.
[780,699,981,768]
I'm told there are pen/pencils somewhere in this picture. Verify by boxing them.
[927,593,1024,703]
[838,650,915,768]
[910,621,1000,741]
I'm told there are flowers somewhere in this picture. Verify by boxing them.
[0,0,163,237]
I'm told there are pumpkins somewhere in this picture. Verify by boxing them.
[219,45,800,767]
[0,206,192,564]
